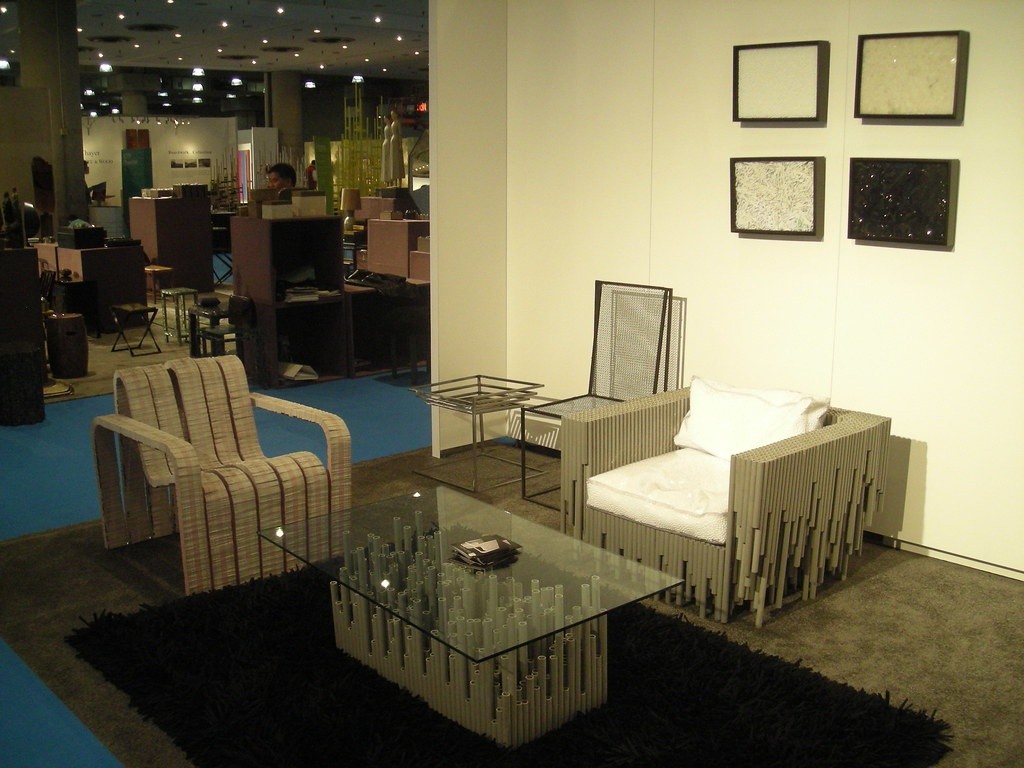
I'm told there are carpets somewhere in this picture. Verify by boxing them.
[64,511,954,768]
[44,279,240,405]
[0,441,1024,768]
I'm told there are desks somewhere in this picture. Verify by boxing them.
[258,484,683,747]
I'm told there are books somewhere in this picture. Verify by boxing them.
[283,287,319,303]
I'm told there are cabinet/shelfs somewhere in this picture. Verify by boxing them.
[230,217,431,389]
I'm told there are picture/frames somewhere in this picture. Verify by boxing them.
[856,30,971,126]
[733,42,830,123]
[730,157,826,236]
[847,156,960,251]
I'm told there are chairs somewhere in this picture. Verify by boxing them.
[519,280,675,510]
[560,391,891,628]
[92,355,351,596]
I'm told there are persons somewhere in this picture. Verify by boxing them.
[306,160,317,190]
[268,163,297,191]
[84,160,97,206]
[390,108,404,188]
[382,114,393,186]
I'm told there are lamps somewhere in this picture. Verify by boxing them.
[352,72,364,83]
[305,78,315,88]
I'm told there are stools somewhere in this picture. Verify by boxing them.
[40,265,266,378]
